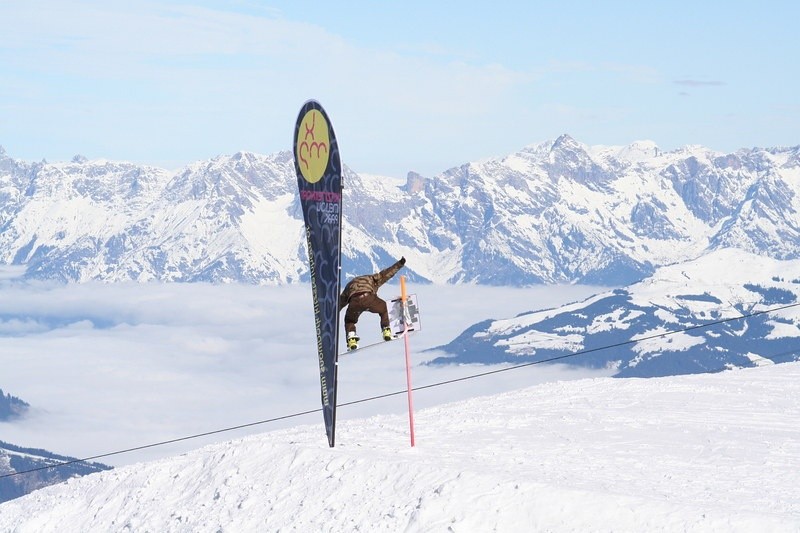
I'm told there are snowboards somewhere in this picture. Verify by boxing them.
[338,336,399,356]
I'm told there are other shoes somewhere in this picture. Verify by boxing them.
[383,328,391,342]
[347,339,357,351]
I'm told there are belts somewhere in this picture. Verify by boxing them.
[350,292,369,301]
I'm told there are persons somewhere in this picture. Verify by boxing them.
[340,257,406,350]
